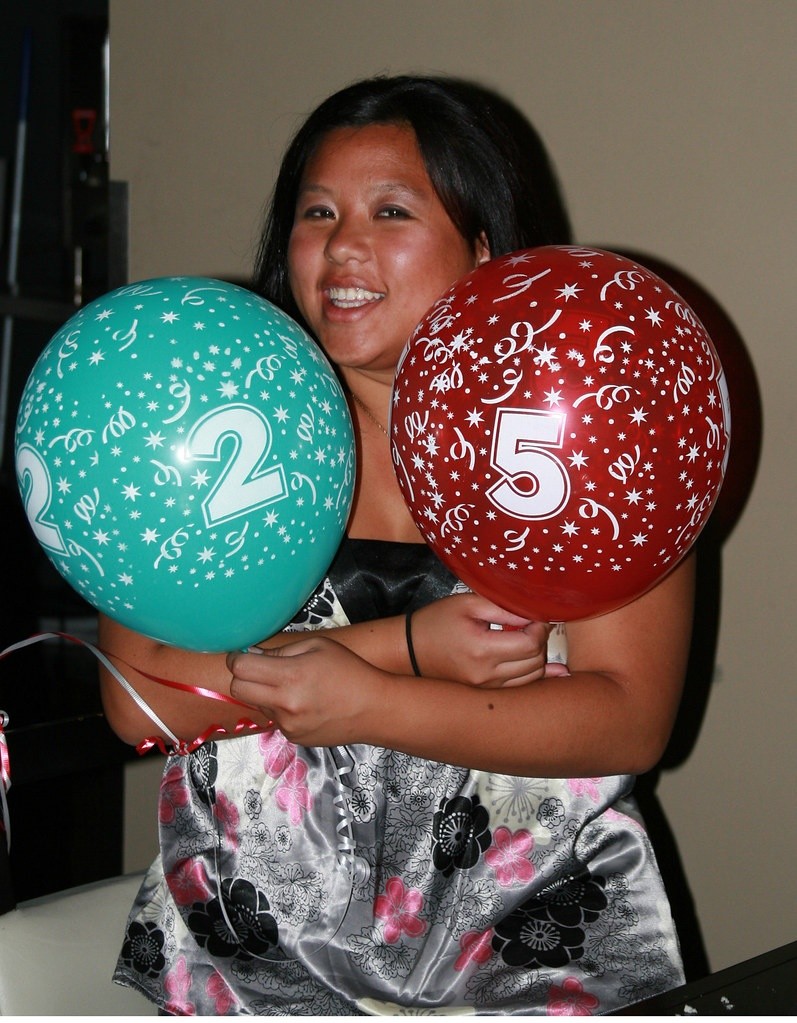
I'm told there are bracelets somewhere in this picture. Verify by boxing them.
[405,609,422,677]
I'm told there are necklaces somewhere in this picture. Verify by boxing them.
[349,394,389,438]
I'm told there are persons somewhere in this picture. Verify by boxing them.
[99,76,698,1015]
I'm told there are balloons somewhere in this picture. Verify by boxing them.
[390,243,733,621]
[15,275,358,651]
